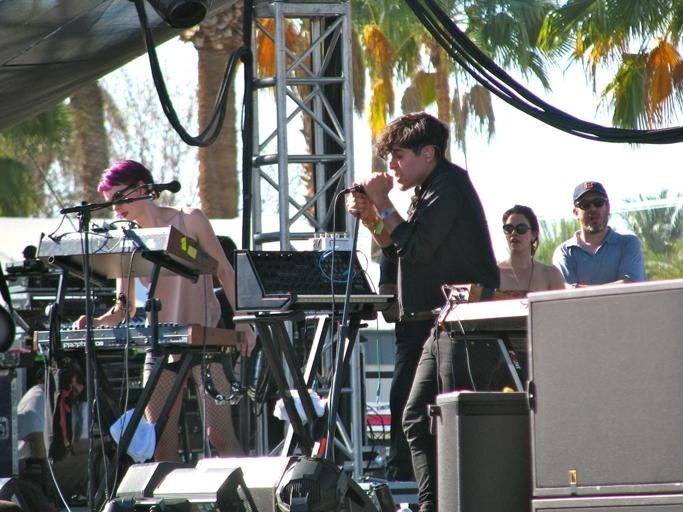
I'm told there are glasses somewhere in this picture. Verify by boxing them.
[576,198,608,210]
[503,224,531,235]
[107,184,133,211]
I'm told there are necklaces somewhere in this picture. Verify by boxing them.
[510,263,535,290]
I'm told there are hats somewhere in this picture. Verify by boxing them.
[574,182,606,203]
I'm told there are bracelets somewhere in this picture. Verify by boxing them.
[365,218,384,235]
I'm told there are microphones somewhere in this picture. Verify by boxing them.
[346,184,366,195]
[145,179,181,195]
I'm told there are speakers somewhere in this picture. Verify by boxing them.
[196,456,298,511]
[149,467,259,512]
[116,461,193,498]
[427,391,530,512]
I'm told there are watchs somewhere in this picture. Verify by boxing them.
[381,207,397,218]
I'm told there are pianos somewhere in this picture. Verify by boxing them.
[233,248,394,312]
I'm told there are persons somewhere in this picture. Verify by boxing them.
[73,159,257,462]
[552,180,645,291]
[380,247,430,483]
[344,113,500,511]
[18,360,81,458]
[497,204,566,386]
